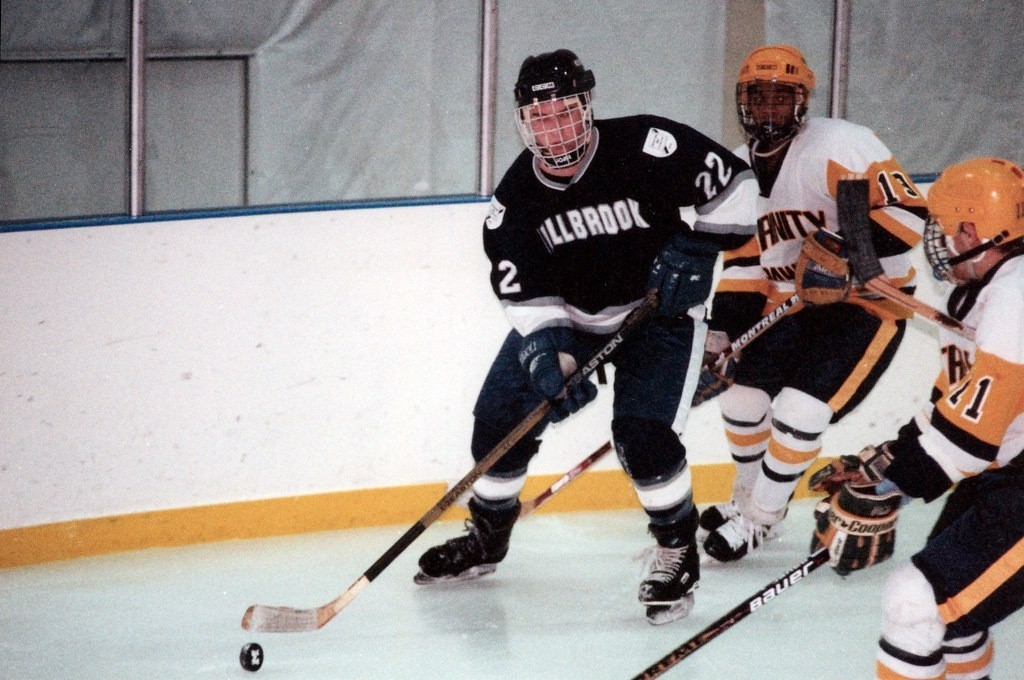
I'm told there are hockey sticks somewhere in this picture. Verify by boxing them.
[628,544,831,680]
[238,284,660,635]
[832,170,978,343]
[445,290,804,520]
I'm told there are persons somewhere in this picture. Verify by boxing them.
[417,49,758,606]
[808,157,1024,679]
[690,45,927,563]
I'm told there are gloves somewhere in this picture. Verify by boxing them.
[795,227,853,307]
[807,443,901,577]
[692,332,736,408]
[650,237,715,317]
[519,326,599,422]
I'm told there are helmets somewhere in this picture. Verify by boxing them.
[515,49,597,169]
[925,157,1024,286]
[737,46,814,146]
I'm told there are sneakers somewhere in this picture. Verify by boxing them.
[700,502,771,562]
[636,506,701,626]
[414,496,522,585]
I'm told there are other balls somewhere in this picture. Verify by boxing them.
[239,640,265,673]
